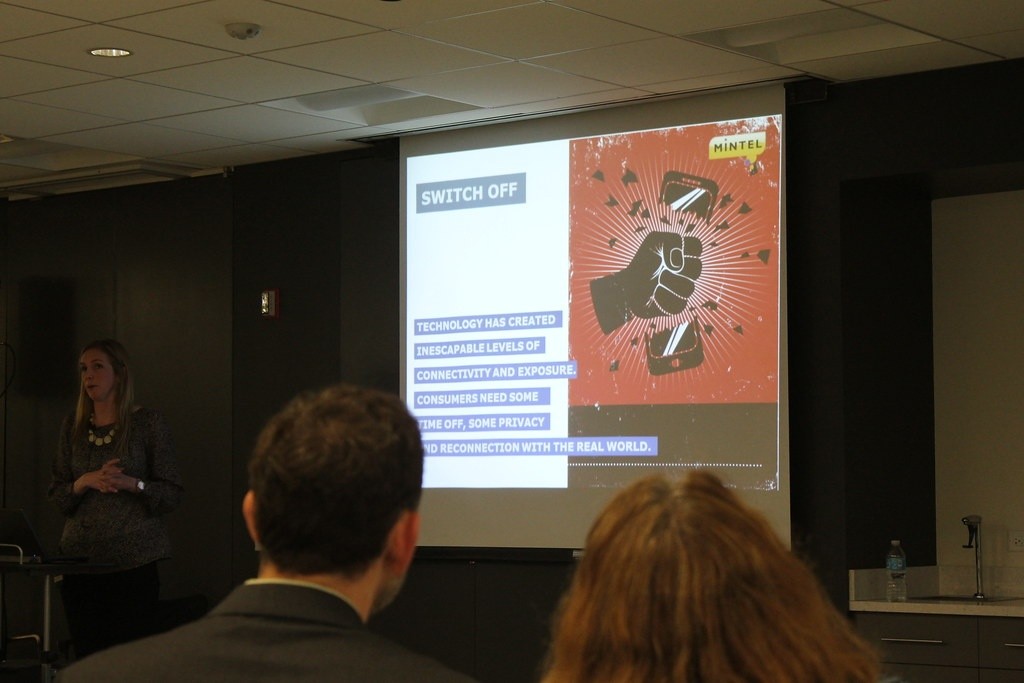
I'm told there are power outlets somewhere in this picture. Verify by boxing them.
[1008,530,1024,553]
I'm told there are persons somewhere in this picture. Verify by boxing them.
[46,339,187,660]
[51,385,485,683]
[544,467,899,683]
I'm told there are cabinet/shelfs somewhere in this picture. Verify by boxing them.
[852,609,1024,683]
[0,556,128,682]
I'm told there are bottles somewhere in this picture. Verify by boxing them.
[886,540,908,603]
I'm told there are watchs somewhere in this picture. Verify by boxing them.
[133,477,145,496]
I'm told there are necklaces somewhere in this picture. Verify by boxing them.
[87,408,119,445]
